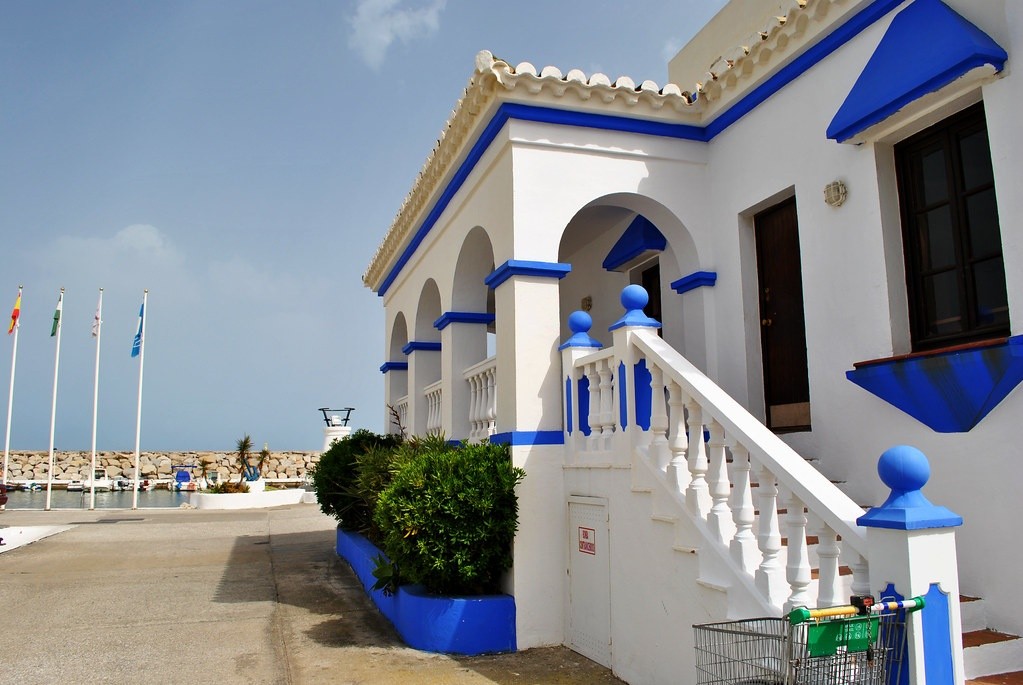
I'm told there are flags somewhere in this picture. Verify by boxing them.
[91,295,104,336]
[130,295,146,358]
[8,290,22,335]
[50,293,63,337]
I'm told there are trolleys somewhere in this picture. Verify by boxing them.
[691,593,925,685]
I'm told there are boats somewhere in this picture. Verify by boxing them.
[0,460,199,494]
